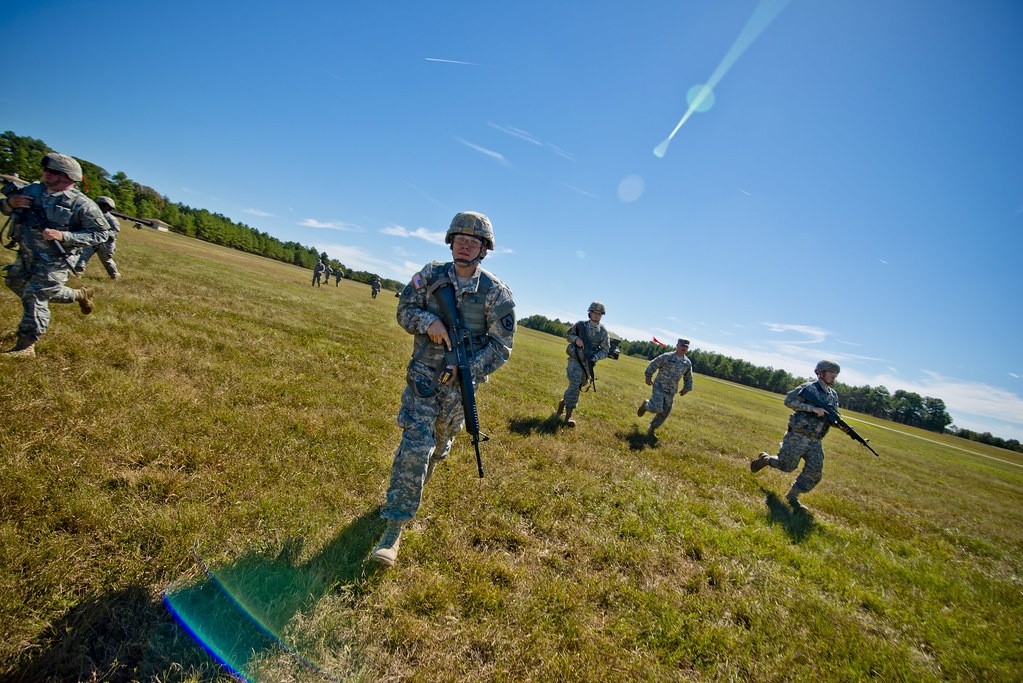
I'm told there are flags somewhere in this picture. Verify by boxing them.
[653,337,665,346]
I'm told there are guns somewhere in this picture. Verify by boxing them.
[797,386,880,457]
[431,281,492,480]
[0,181,79,276]
[575,321,597,393]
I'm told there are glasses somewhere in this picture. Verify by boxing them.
[453,234,482,248]
[43,167,67,179]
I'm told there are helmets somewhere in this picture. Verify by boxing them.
[318,259,321,263]
[339,268,342,271]
[40,152,82,182]
[376,277,381,281]
[588,302,605,316]
[93,196,116,211]
[814,360,840,376]
[445,211,495,251]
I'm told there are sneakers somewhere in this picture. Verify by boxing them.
[111,271,121,281]
[68,269,82,280]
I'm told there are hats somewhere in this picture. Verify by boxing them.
[678,339,690,348]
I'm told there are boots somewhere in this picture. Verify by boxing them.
[563,409,576,427]
[750,450,770,473]
[372,520,404,568]
[0,336,36,359]
[646,426,659,441]
[557,398,565,415]
[424,460,437,484]
[785,486,809,511]
[71,286,95,314]
[637,399,649,417]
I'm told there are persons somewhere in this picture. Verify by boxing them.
[637,338,693,440]
[132,221,143,230]
[558,302,609,428]
[751,360,854,511]
[0,154,122,359]
[370,211,516,567]
[312,259,344,288]
